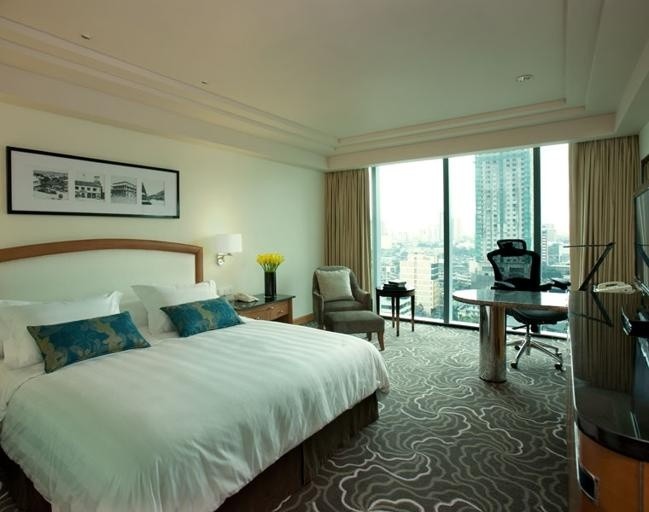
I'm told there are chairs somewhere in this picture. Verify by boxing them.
[487,238,571,369]
[311,265,374,342]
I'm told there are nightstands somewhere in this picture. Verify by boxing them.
[227,292,296,324]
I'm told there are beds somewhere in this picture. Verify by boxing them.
[1,241,389,512]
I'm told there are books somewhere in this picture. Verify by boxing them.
[382,277,407,292]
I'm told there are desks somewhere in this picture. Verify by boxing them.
[452,288,570,382]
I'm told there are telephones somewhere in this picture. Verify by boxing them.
[592,281,634,294]
[232,291,259,303]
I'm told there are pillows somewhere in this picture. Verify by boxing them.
[159,293,246,337]
[26,311,152,373]
[315,269,355,302]
[0,290,122,370]
[131,281,226,334]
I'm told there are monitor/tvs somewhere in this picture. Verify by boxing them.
[621,185,649,323]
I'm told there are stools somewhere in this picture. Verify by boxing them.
[325,310,385,351]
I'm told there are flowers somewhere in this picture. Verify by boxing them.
[256,251,285,272]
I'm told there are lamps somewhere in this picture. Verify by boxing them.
[214,233,242,266]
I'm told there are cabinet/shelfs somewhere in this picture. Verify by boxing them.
[567,289,649,509]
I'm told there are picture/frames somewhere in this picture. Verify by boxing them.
[5,144,181,220]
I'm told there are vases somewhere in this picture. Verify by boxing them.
[264,272,276,299]
